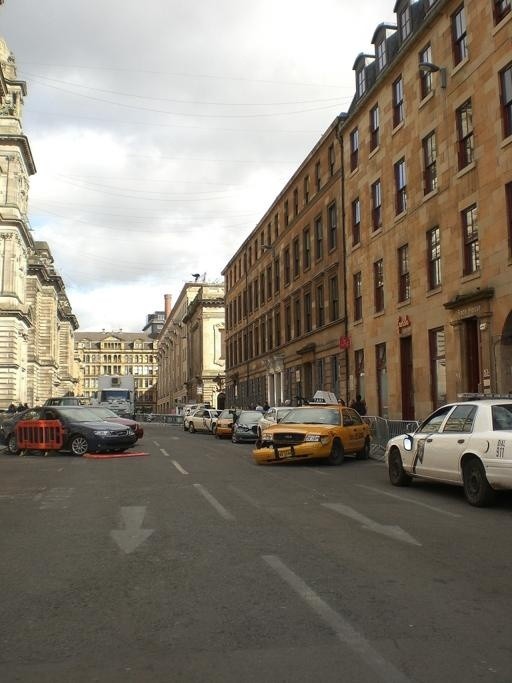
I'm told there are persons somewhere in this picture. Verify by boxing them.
[8,401,29,413]
[229,394,367,418]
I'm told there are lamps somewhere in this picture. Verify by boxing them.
[260,244,274,258]
[419,63,447,88]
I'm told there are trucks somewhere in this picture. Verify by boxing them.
[98,375,134,420]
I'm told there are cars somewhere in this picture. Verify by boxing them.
[385,393,512,505]
[252,390,372,464]
[182,404,291,443]
[0,397,143,456]
